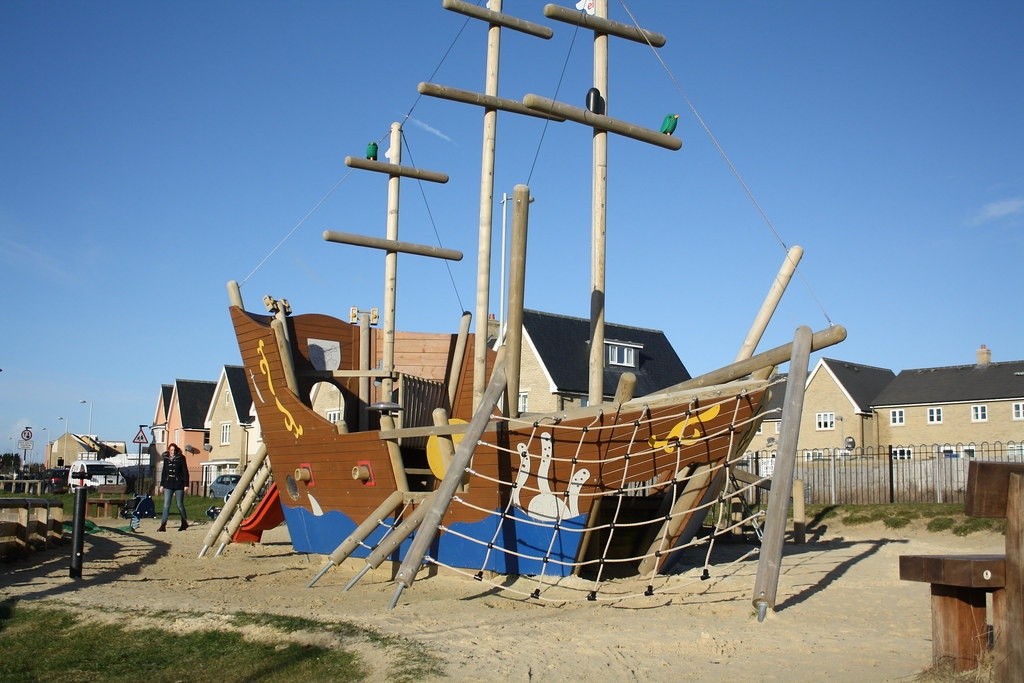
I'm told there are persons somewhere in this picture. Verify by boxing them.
[156,443,190,533]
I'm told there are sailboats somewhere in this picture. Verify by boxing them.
[227,0,850,583]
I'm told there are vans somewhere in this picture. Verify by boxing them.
[67,459,127,496]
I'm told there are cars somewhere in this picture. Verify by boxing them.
[0,463,71,496]
[209,474,243,500]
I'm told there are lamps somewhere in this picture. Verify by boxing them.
[835,416,843,421]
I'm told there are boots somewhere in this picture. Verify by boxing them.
[178,520,188,531]
[157,522,166,532]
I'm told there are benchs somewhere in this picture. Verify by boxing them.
[897,461,1024,683]
[84,484,126,518]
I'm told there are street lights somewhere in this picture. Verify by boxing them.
[41,428,51,469]
[58,416,68,467]
[80,400,93,460]
